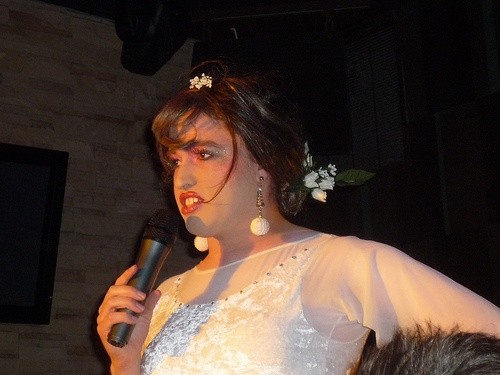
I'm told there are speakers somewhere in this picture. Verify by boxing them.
[114,0,194,76]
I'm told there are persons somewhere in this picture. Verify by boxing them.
[94,78,500,375]
[356,329,500,375]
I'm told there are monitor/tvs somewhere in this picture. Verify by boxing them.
[0,142,69,324]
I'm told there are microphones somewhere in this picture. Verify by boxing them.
[106,208,184,349]
